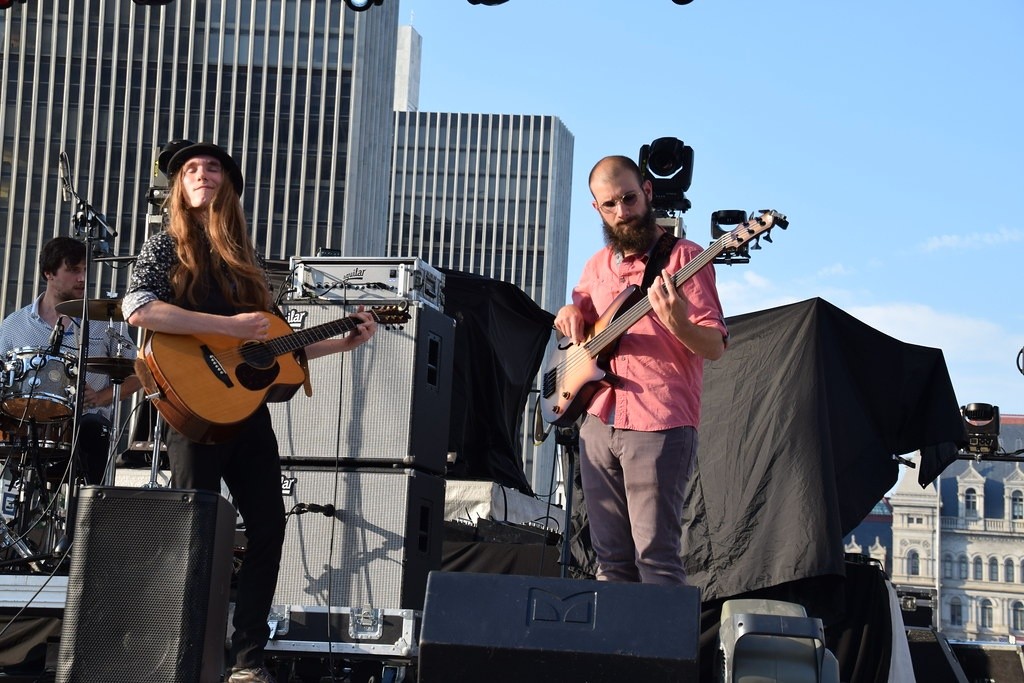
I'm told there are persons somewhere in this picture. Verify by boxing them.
[554,156,727,587]
[120,139,377,683]
[1,237,142,485]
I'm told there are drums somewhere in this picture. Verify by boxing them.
[1,347,78,425]
[0,414,72,462]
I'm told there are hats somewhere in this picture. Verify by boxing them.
[166,144,244,198]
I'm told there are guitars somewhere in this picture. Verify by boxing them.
[134,303,413,444]
[540,209,789,427]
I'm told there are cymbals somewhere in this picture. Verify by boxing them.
[55,298,124,322]
[77,356,136,378]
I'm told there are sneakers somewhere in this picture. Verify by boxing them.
[229,665,274,683]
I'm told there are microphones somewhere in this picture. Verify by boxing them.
[48,317,63,343]
[59,154,70,202]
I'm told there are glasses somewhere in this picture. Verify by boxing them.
[595,191,646,215]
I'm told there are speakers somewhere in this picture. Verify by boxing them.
[420,568,702,683]
[56,481,236,683]
